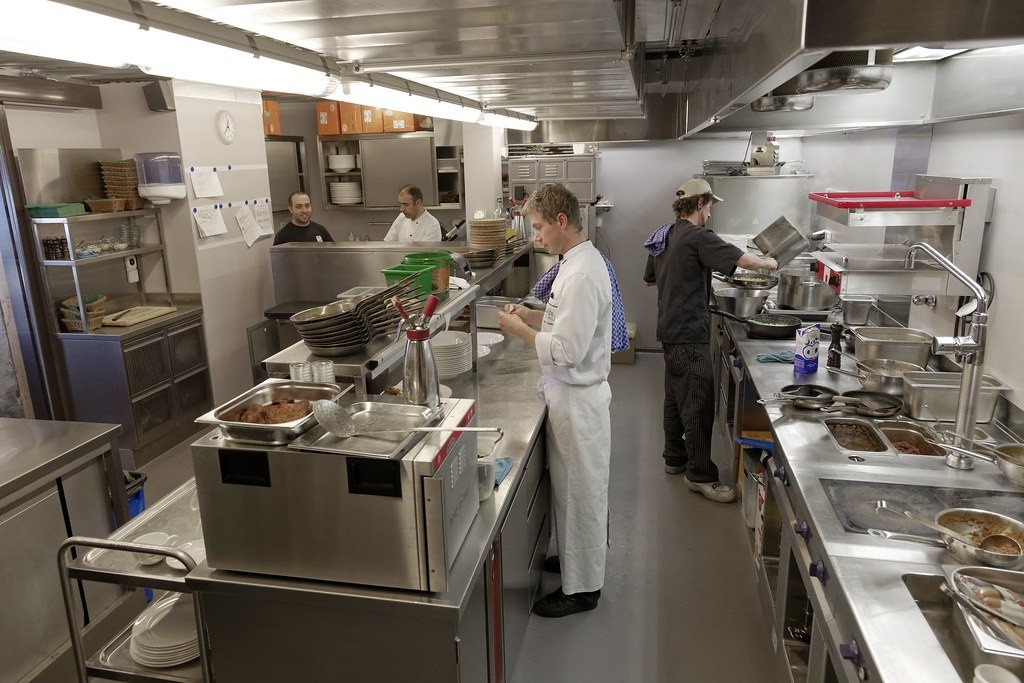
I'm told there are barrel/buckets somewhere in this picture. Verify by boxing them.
[403,252,452,301]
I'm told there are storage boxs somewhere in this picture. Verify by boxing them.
[383,109,434,133]
[611,323,638,364]
[736,430,774,495]
[262,101,280,135]
[338,100,363,135]
[315,102,340,135]
[361,106,383,133]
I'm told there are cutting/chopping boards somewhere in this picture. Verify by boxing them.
[100,305,177,327]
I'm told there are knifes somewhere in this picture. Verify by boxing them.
[112,309,131,322]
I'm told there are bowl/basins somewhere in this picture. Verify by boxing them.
[73,223,144,259]
[462,234,529,268]
[714,288,772,320]
[429,325,505,378]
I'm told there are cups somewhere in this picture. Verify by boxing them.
[132,532,179,566]
[289,360,336,386]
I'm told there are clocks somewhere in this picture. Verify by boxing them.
[215,110,237,145]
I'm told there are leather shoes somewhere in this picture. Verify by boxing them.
[533,585,601,618]
[544,555,560,573]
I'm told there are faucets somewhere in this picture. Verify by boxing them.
[905,241,986,313]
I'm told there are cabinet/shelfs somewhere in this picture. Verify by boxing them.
[32,206,174,333]
[316,136,468,210]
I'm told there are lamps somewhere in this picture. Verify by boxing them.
[0,0,539,131]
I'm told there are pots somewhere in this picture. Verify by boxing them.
[289,271,435,357]
[757,354,925,416]
[701,216,838,337]
[870,442,1024,562]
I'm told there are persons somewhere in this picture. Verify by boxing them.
[498,184,613,617]
[643,178,778,503]
[273,191,335,246]
[383,184,442,242]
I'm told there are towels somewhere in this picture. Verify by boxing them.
[643,221,676,257]
[530,249,630,354]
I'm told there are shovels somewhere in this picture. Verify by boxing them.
[832,396,896,410]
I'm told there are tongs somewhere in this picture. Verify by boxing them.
[941,583,1024,648]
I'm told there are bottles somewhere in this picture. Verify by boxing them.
[134,152,187,205]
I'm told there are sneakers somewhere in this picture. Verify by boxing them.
[684,473,736,503]
[663,460,684,475]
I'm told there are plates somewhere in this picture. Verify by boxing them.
[165,540,207,569]
[436,158,461,170]
[327,153,363,206]
[128,594,210,667]
[466,219,508,255]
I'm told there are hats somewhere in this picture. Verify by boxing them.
[675,179,723,204]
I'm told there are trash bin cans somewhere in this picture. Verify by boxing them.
[105,469,154,602]
